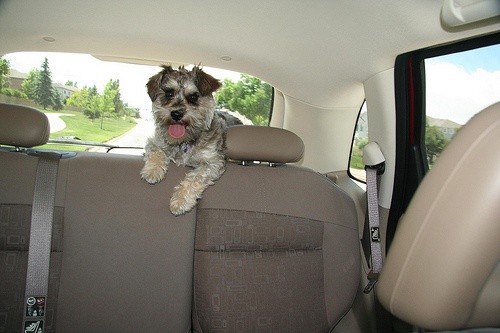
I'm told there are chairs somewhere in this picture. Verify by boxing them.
[375,100,499,333]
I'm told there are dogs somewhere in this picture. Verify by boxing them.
[140,61,254,217]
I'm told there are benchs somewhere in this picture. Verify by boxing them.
[0,101,364,333]
